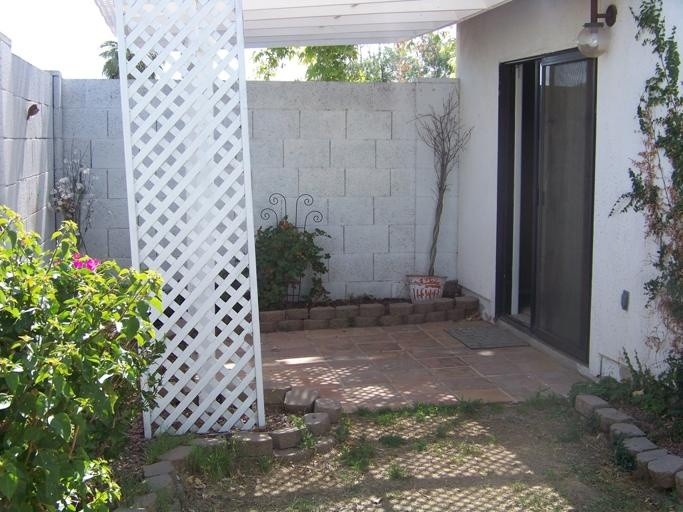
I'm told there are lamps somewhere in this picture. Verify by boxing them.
[575,0,617,60]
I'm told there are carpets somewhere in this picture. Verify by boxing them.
[444,323,529,350]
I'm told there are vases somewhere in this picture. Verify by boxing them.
[405,274,448,301]
